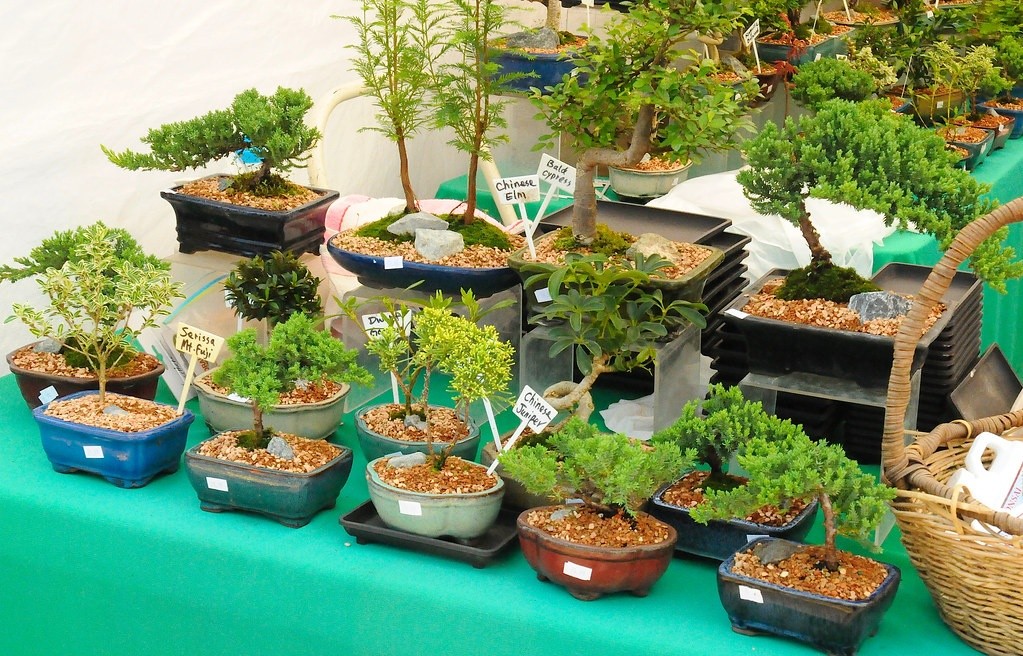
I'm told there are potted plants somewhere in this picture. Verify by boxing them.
[0,0,1023,656]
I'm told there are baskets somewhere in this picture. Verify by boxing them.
[881,197,1023,656]
